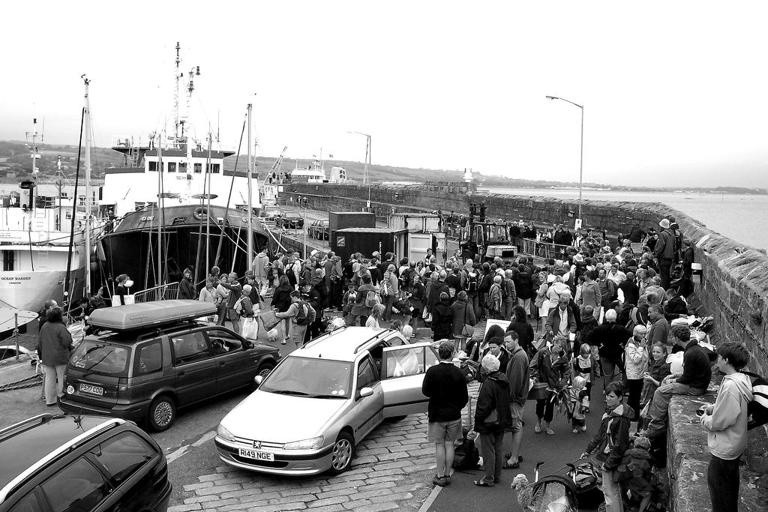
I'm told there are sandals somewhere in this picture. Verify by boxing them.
[473,480,490,487]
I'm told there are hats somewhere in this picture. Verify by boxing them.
[310,250,321,257]
[372,251,380,257]
[659,219,670,229]
[243,284,252,292]
[352,263,361,273]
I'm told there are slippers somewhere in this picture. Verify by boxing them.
[545,429,555,435]
[535,425,542,433]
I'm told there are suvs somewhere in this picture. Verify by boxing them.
[0,413,172,512]
[57,300,282,431]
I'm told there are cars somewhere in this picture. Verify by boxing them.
[308,218,329,240]
[276,211,304,228]
[0,345,29,363]
[214,327,441,476]
[260,206,281,220]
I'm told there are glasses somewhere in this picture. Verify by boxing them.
[559,301,568,305]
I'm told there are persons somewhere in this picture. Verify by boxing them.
[473,354,512,486]
[643,326,712,436]
[618,272,639,306]
[696,343,754,512]
[591,308,631,393]
[581,305,601,378]
[596,269,614,309]
[37,307,73,407]
[389,320,401,331]
[438,202,509,243]
[640,276,668,304]
[647,305,669,365]
[476,337,510,383]
[401,324,413,346]
[503,330,529,469]
[215,274,231,326]
[421,343,468,486]
[620,436,654,511]
[610,299,624,325]
[577,272,602,310]
[449,290,476,353]
[670,217,679,239]
[91,278,114,307]
[535,272,549,339]
[573,343,600,401]
[240,284,258,341]
[501,269,516,321]
[251,246,269,302]
[301,282,321,343]
[381,264,398,323]
[271,274,294,344]
[680,239,694,281]
[39,300,60,402]
[209,265,220,288]
[514,264,534,318]
[509,221,537,246]
[488,276,502,319]
[536,223,660,285]
[198,277,217,323]
[275,290,316,349]
[426,271,440,299]
[564,377,589,433]
[268,169,292,184]
[271,248,464,310]
[640,342,671,431]
[529,336,571,435]
[546,274,572,314]
[445,267,462,301]
[663,288,688,321]
[366,303,386,327]
[216,273,242,335]
[77,207,115,235]
[427,270,450,338]
[461,259,481,326]
[342,283,357,327]
[478,263,494,321]
[580,380,635,511]
[655,218,674,291]
[296,196,302,208]
[624,324,648,421]
[303,196,308,208]
[179,268,196,299]
[351,275,376,326]
[506,306,534,353]
[540,295,577,348]
[410,275,425,338]
[637,292,657,325]
[247,275,260,328]
[75,296,94,325]
[430,292,452,342]
[494,256,538,277]
[116,273,128,305]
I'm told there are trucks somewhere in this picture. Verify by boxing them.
[333,227,432,267]
[329,211,375,247]
[389,213,447,266]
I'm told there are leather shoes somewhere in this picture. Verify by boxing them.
[503,462,520,469]
[505,453,523,462]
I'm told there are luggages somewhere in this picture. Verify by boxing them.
[452,395,479,471]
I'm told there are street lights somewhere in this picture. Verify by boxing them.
[346,128,370,208]
[545,94,584,224]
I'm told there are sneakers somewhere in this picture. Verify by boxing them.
[572,429,578,433]
[285,335,291,340]
[580,424,587,431]
[444,475,452,485]
[432,474,447,487]
[643,428,665,437]
[280,340,287,344]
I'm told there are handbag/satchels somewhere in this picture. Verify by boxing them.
[242,317,258,340]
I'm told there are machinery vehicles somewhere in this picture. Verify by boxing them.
[458,203,517,265]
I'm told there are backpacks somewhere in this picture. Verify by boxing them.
[292,301,315,325]
[740,371,768,430]
[462,269,479,297]
[233,295,248,315]
[596,280,618,302]
[285,262,297,286]
[528,348,549,400]
